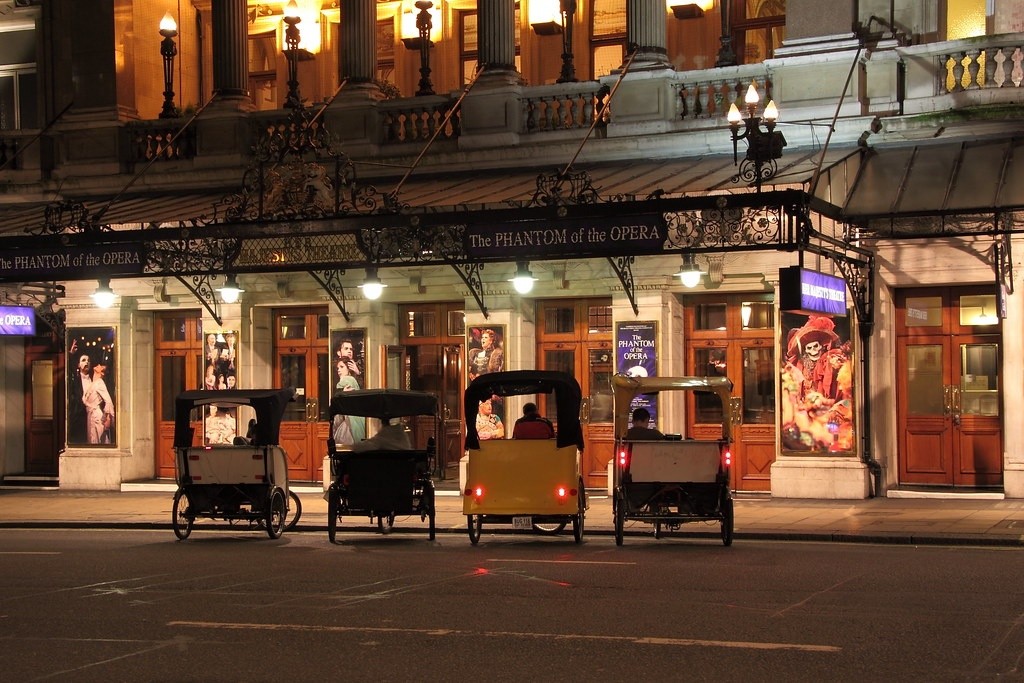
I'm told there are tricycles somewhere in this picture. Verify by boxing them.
[172,389,302,539]
[327,389,438,542]
[463,370,586,544]
[611,372,734,546]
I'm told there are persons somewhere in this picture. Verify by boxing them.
[206,350,235,390]
[476,400,504,439]
[333,339,365,443]
[68,339,114,444]
[351,417,411,450]
[221,334,236,349]
[206,334,221,356]
[513,403,556,439]
[205,406,236,443]
[234,419,258,445]
[469,329,504,380]
[626,408,665,440]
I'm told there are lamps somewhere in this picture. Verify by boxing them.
[158,9,178,117]
[671,253,707,288]
[728,81,779,193]
[284,0,302,107]
[89,279,119,309]
[356,268,388,300]
[507,260,539,294]
[215,274,245,304]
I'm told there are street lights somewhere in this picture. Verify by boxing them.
[728,87,779,189]
[285,1,302,103]
[156,11,182,119]
